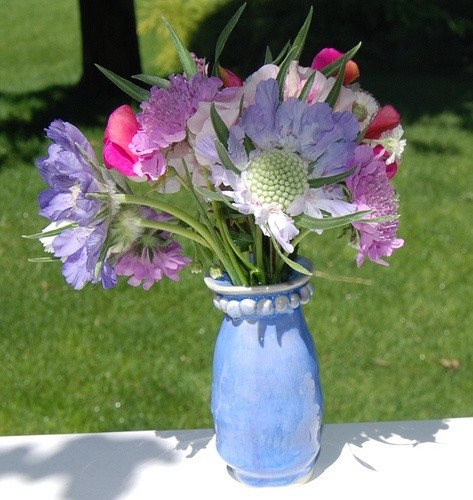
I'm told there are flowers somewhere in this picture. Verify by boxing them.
[21,2,409,291]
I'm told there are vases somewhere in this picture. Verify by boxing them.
[200,252,326,489]
